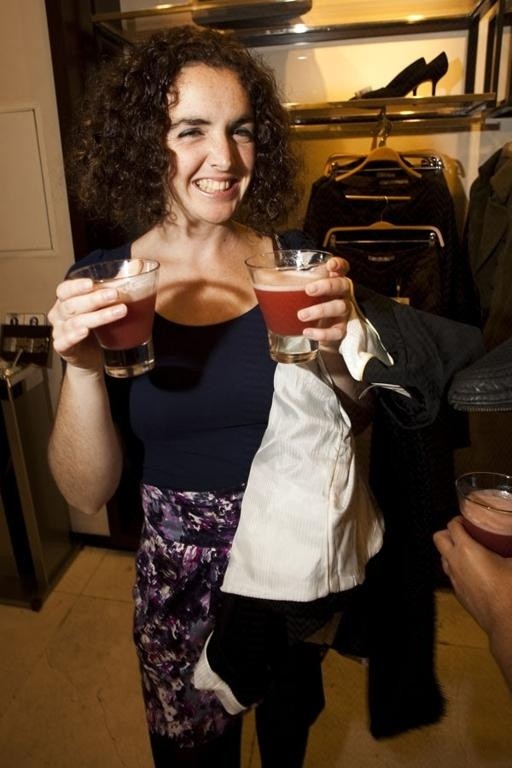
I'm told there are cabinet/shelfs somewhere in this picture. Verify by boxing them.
[93,0,509,141]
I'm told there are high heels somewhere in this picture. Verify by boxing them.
[347,52,448,99]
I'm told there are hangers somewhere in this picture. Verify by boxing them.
[318,137,454,257]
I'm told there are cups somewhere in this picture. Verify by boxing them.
[244,249,333,365]
[64,257,161,378]
[455,471,511,556]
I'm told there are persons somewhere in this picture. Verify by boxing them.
[433,515,512,696]
[47,24,373,768]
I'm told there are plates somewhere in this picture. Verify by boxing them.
[193,0,315,29]
